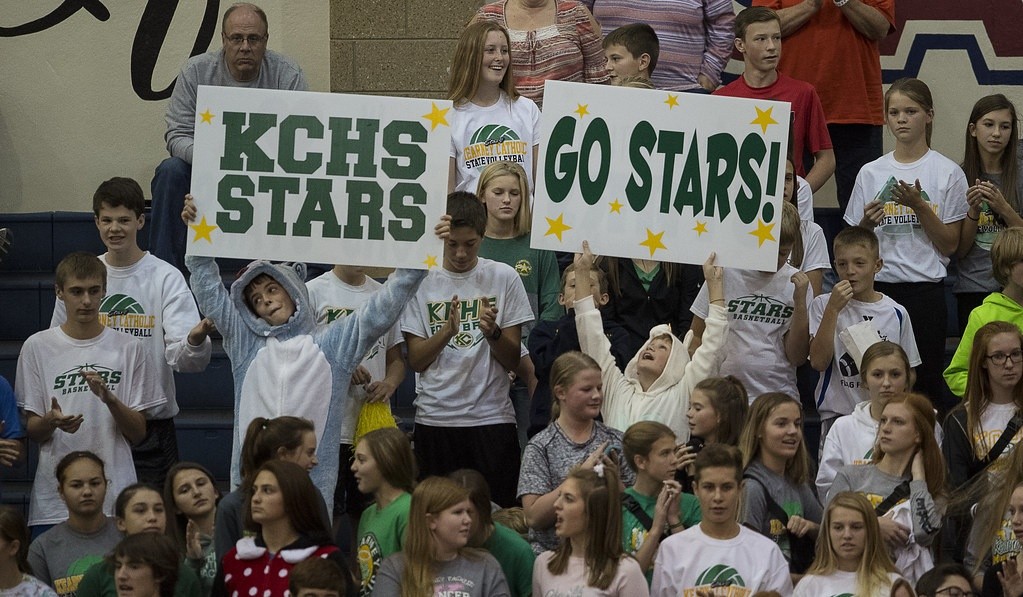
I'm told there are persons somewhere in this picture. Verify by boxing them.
[0,0,1023,597]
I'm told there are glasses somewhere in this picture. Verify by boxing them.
[936,585,974,597]
[223,32,266,45]
[985,350,1023,366]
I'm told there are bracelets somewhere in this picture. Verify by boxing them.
[967,211,980,221]
[710,299,725,303]
[670,521,683,529]
[833,0,849,6]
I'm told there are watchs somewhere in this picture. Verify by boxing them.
[482,323,502,340]
